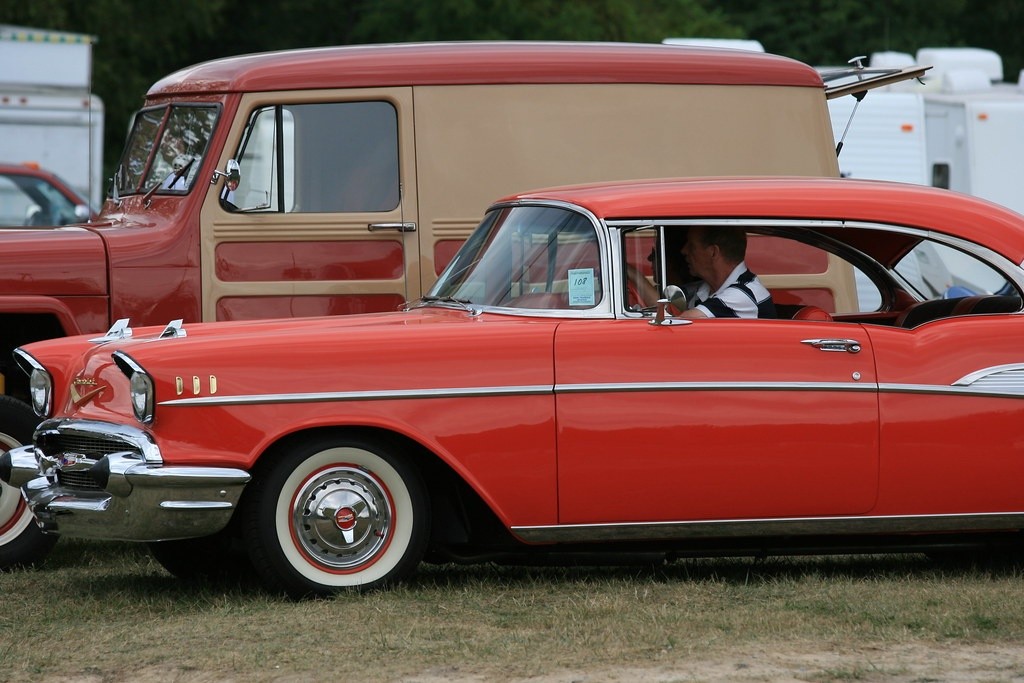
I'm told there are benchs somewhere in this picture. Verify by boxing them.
[894,294,1023,329]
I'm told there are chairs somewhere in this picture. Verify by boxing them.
[769,304,832,322]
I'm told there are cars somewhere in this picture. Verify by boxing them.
[0,162,99,229]
[0,176,1024,602]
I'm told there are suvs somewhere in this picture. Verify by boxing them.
[0,37,933,599]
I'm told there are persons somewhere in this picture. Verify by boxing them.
[623,226,779,321]
